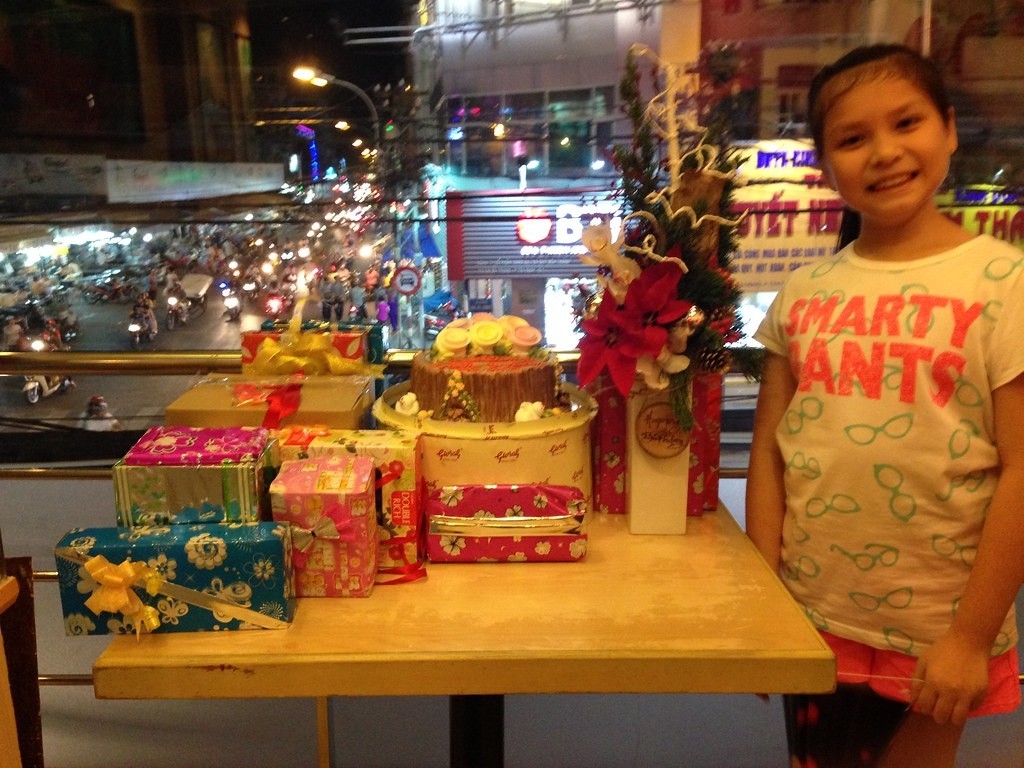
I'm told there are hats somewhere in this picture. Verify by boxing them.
[5,315,14,320]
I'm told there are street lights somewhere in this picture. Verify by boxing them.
[292,64,381,151]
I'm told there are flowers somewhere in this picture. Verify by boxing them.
[562,37,766,431]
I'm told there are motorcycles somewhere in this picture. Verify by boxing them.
[0,265,130,339]
[167,286,207,331]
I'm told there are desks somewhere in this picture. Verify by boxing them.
[92,500,840,768]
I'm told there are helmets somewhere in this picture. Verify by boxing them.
[46,320,53,324]
[90,396,106,410]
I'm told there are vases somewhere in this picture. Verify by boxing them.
[623,372,693,537]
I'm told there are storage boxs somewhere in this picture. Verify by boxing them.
[54,317,723,637]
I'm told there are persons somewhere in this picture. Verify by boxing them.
[0,203,401,431]
[747,41,1024,767]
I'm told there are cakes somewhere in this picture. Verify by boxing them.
[395,310,568,424]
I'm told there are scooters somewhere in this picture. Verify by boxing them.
[127,244,199,305]
[17,333,77,405]
[401,299,471,341]
[130,303,159,347]
[218,225,368,325]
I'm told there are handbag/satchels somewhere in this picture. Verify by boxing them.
[20,336,29,350]
